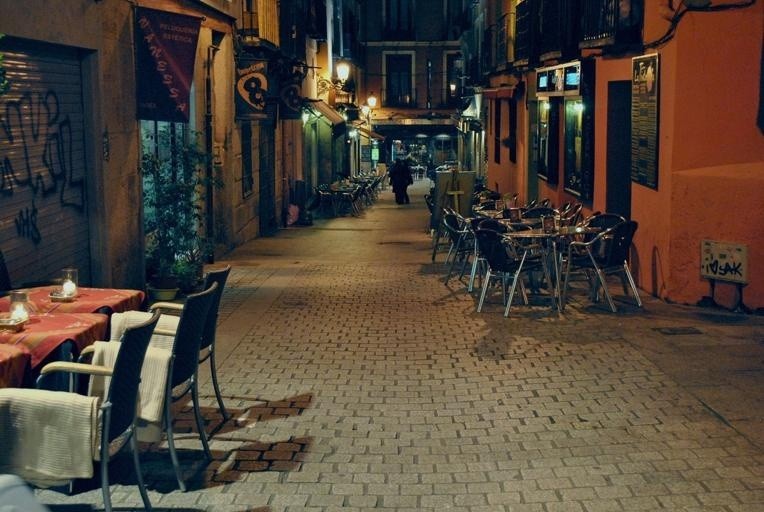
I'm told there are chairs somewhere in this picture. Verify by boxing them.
[315,171,386,217]
[0,307,162,511]
[145,263,233,428]
[80,280,220,493]
[439,192,642,318]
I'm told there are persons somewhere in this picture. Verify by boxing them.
[389,157,404,204]
[398,159,414,204]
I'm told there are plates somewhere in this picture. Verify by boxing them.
[0,320,24,334]
[48,292,75,302]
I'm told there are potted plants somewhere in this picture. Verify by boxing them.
[138,126,225,302]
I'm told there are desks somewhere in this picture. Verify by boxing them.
[4,282,144,342]
[2,312,104,395]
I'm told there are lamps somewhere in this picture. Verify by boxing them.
[317,56,349,97]
[368,92,376,113]
